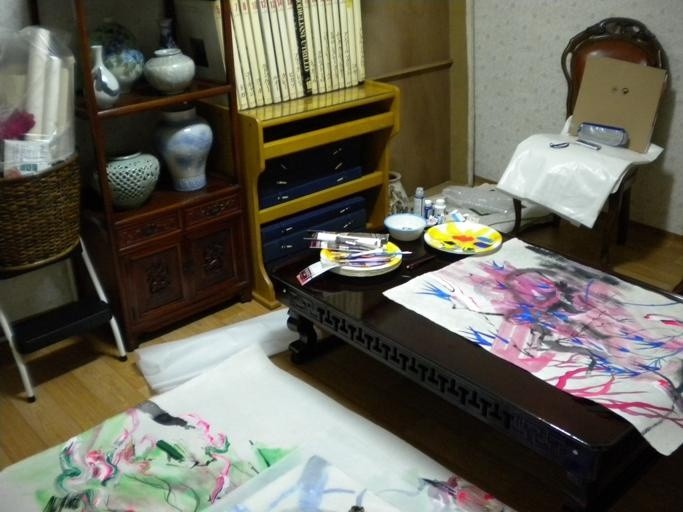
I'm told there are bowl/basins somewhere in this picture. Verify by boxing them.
[384,214,426,241]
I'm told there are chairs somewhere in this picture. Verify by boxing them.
[508,15,673,261]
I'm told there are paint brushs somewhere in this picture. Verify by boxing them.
[302,236,413,263]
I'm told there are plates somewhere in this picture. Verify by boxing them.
[423,221,503,255]
[320,240,402,277]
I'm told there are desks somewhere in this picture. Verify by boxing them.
[265,216,682,508]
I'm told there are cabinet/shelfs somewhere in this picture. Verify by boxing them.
[23,0,255,351]
[237,78,402,311]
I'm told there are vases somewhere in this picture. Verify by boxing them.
[386,168,411,217]
